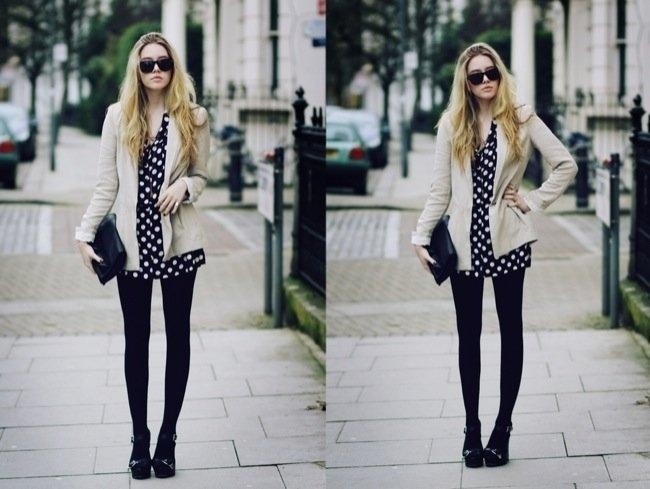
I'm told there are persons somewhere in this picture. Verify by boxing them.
[408,42,580,469]
[72,31,213,482]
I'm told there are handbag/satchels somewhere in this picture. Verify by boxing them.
[91,214,127,284]
[429,220,457,285]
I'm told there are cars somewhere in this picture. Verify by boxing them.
[0,98,40,163]
[326,102,383,196]
[0,114,21,189]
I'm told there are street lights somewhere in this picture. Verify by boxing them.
[398,50,420,178]
[48,35,69,171]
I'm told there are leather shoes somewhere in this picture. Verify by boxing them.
[462,424,484,468]
[485,424,512,467]
[153,432,178,478]
[129,431,152,478]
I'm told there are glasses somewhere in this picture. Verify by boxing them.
[467,67,500,85]
[139,57,171,74]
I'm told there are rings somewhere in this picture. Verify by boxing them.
[175,202,180,206]
